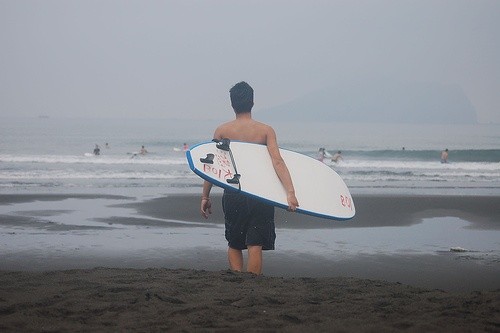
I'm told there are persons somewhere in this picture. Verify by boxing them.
[322,148,328,158]
[183,142,188,151]
[441,148,449,162]
[201,81,299,275]
[94,145,101,155]
[318,148,324,160]
[331,151,344,162]
[105,143,110,150]
[140,145,147,154]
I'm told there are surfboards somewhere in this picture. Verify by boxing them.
[185,138,357,221]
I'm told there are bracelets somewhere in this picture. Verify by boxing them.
[202,193,210,200]
